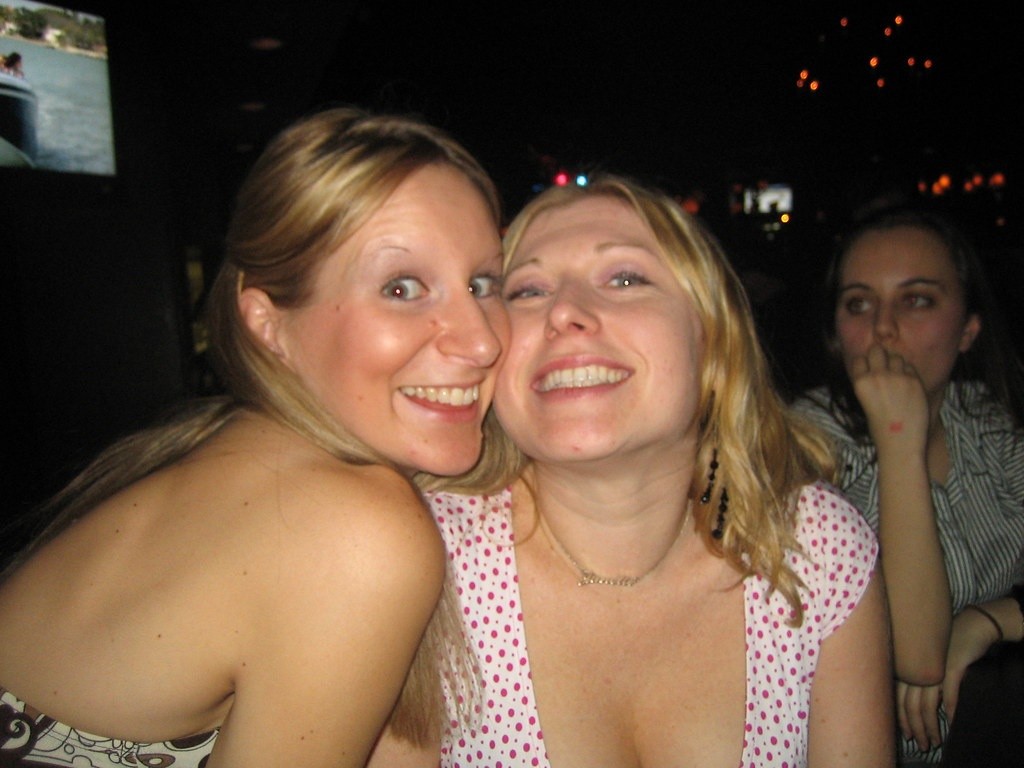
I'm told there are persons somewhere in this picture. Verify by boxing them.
[783,203,1023,767]
[368,169,900,768]
[1,107,513,768]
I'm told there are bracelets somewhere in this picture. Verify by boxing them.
[964,602,1005,643]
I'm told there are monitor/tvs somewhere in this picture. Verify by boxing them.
[0,0,119,178]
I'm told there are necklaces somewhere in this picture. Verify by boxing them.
[518,476,688,589]
